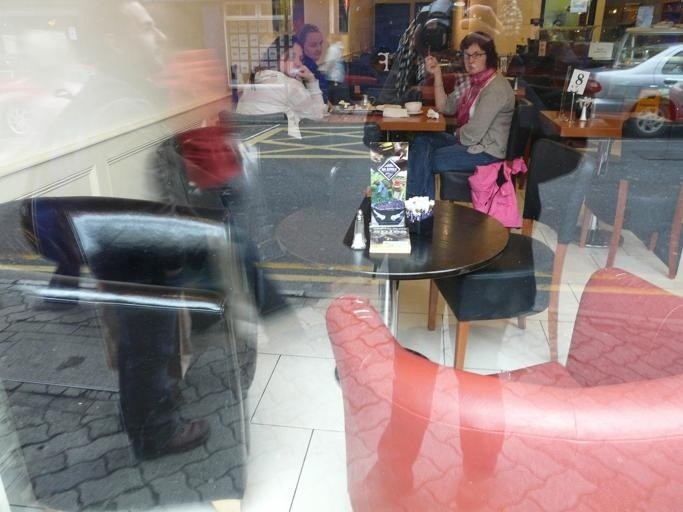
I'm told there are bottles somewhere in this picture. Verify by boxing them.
[578,103,588,121]
[349,208,368,251]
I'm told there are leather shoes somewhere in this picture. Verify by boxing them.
[134,421,209,459]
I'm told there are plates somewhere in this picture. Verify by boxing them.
[375,104,402,112]
[406,109,423,115]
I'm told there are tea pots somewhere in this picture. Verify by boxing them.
[359,94,376,108]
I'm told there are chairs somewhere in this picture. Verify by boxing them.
[212,39,682,236]
[576,116,682,280]
[428,137,594,370]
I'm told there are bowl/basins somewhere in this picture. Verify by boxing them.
[404,101,422,113]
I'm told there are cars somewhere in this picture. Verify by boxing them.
[0,68,59,142]
[576,38,682,140]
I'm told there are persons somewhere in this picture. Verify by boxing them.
[296,23,331,102]
[547,20,563,42]
[360,1,595,510]
[318,32,356,114]
[406,31,516,201]
[235,34,324,140]
[63,2,213,457]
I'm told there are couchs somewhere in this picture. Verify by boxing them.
[153,123,344,315]
[324,264,683,512]
[1,191,261,511]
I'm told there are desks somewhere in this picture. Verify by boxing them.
[274,196,510,386]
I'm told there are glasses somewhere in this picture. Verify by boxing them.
[463,52,486,59]
